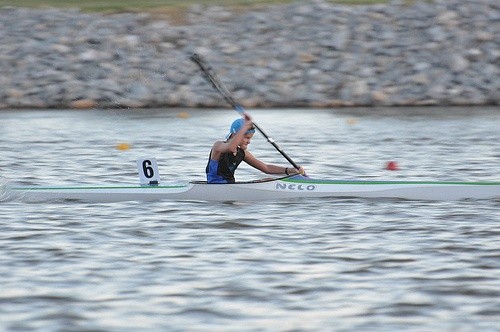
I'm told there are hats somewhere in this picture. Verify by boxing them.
[225,118,255,140]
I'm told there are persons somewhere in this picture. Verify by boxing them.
[206,113,305,184]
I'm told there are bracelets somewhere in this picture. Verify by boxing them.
[285,168,289,175]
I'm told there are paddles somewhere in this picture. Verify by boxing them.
[190,51,308,178]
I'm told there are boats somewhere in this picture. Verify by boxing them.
[0,174,500,203]
